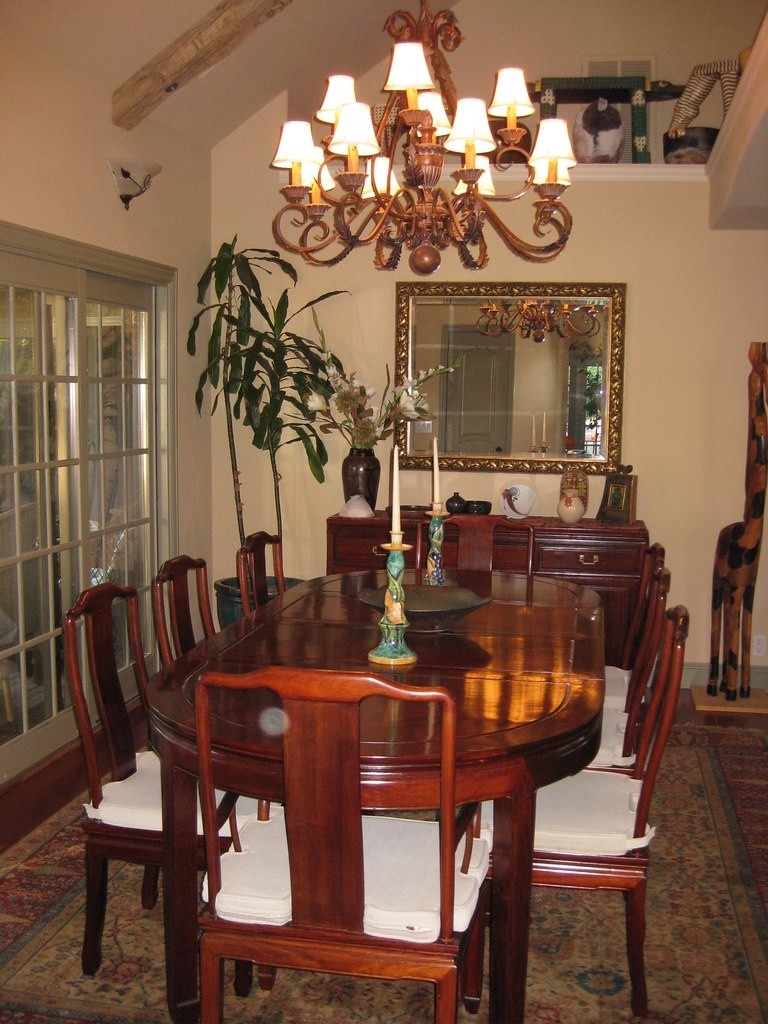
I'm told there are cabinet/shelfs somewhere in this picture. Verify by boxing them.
[327,508,648,664]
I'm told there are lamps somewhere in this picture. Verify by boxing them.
[106,159,163,211]
[476,300,600,342]
[269,1,576,274]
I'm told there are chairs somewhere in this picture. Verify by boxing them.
[194,666,490,1023]
[416,514,535,577]
[65,582,265,999]
[458,606,689,1019]
[236,530,286,614]
[152,556,283,818]
[587,543,672,773]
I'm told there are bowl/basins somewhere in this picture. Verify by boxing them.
[359,584,494,633]
[465,501,492,514]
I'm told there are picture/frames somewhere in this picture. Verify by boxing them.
[596,474,637,524]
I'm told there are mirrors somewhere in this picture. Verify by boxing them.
[395,283,626,475]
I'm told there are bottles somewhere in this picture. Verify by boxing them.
[446,492,466,513]
[557,489,584,524]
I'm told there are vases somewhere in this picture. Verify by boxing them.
[341,448,381,511]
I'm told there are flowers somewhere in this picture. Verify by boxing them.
[283,304,465,449]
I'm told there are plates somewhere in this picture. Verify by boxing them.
[385,505,431,518]
[499,484,538,519]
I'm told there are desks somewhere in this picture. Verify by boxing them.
[142,570,606,1024]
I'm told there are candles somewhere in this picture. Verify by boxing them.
[391,443,402,531]
[433,437,439,502]
[543,412,546,442]
[532,415,535,446]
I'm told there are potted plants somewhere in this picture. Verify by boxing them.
[189,236,353,631]
